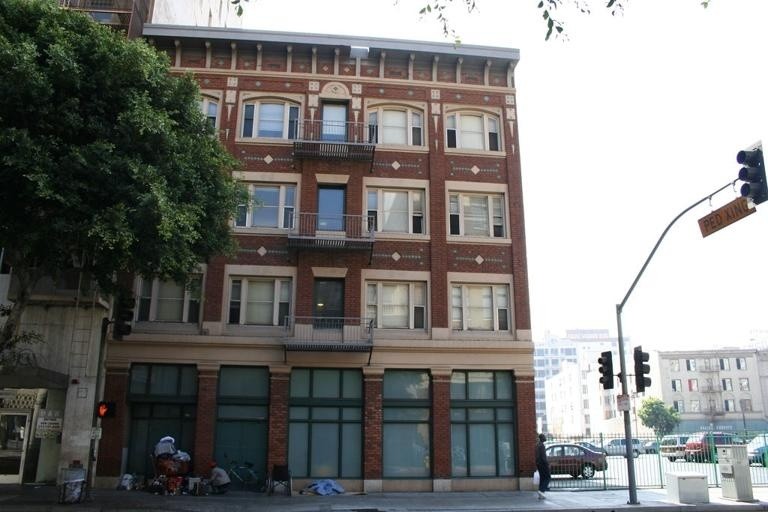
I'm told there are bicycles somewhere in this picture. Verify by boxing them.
[218,453,270,494]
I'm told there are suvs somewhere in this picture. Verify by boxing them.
[595,437,646,460]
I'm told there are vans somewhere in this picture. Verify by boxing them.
[682,429,732,464]
[657,433,691,462]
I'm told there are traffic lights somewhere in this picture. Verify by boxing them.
[111,290,137,341]
[597,350,614,390]
[96,399,116,419]
[634,345,651,392]
[735,140,768,205]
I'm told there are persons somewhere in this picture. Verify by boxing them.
[535,433,551,499]
[205,461,231,494]
[155,438,178,495]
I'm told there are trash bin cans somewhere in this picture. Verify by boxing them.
[57,467,87,505]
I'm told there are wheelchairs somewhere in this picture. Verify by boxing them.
[268,462,293,497]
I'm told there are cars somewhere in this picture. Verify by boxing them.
[747,433,767,466]
[642,440,658,454]
[544,443,608,481]
[573,440,608,455]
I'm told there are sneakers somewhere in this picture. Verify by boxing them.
[537,489,547,500]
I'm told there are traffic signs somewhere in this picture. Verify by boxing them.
[698,193,757,239]
